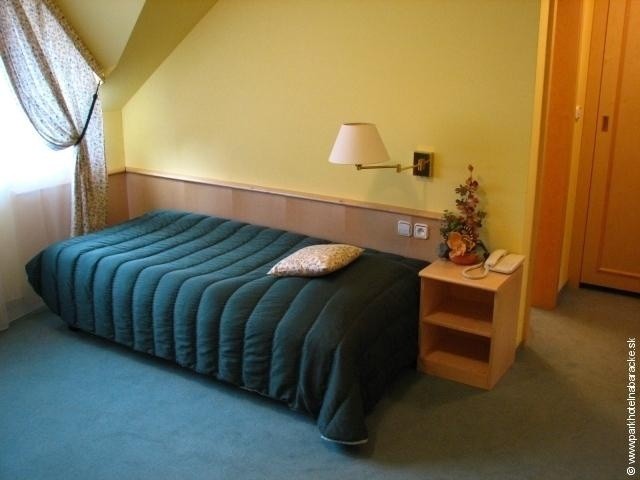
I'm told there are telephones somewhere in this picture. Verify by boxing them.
[484,248,526,274]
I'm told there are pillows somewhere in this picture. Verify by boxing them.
[267,244,366,277]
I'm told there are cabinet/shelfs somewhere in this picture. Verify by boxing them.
[417,257,523,390]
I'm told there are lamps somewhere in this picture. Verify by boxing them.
[327,123,433,178]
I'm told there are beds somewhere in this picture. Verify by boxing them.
[26,208,431,446]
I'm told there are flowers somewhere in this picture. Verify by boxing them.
[440,165,487,257]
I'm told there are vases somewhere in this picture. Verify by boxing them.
[449,245,485,265]
